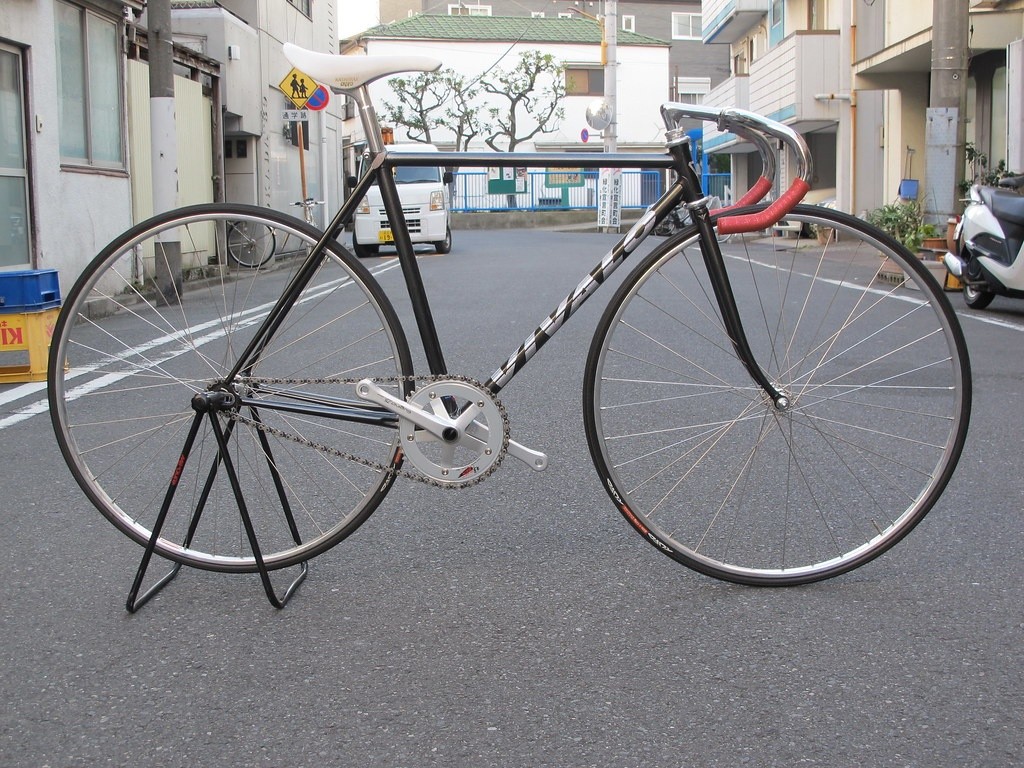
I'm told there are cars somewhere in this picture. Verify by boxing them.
[795,199,835,239]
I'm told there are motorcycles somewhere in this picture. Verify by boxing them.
[943,183,1024,310]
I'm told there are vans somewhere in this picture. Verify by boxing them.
[352,146,451,256]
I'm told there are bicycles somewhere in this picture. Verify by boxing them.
[651,196,738,244]
[954,175,1023,262]
[221,213,277,270]
[288,198,329,263]
[44,43,976,614]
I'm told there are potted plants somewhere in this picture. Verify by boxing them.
[816,225,835,246]
[868,200,932,286]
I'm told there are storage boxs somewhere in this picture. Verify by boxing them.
[0,267,72,387]
